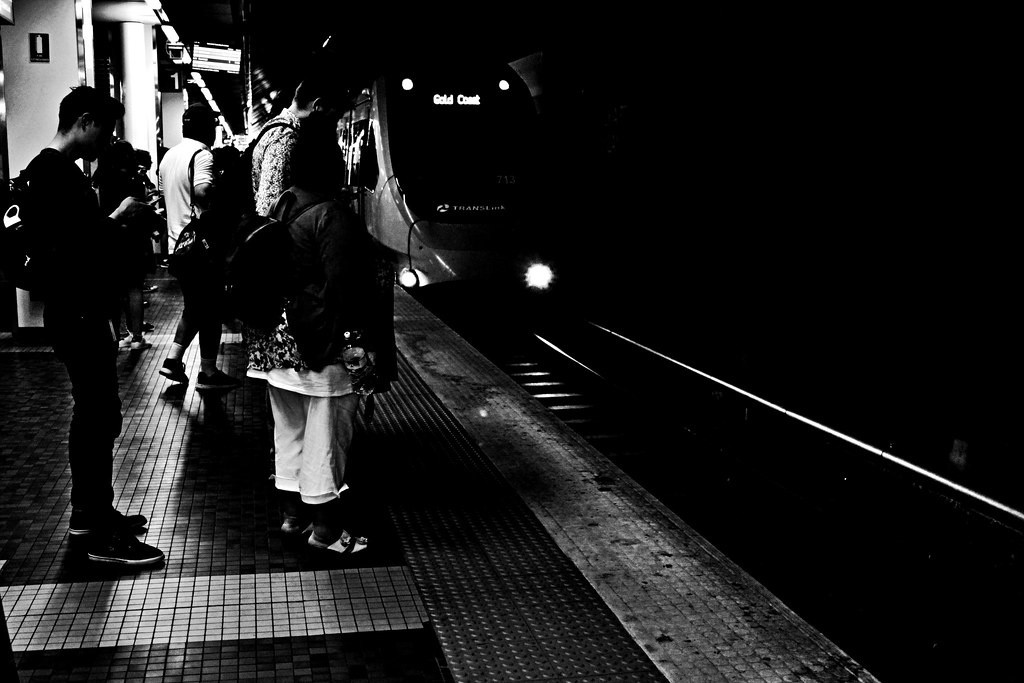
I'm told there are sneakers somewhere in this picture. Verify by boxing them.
[196,369,242,389]
[68,504,148,535]
[130,338,146,349]
[159,357,190,384]
[89,523,165,566]
[119,335,133,348]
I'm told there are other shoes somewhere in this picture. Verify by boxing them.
[308,528,368,554]
[278,512,337,535]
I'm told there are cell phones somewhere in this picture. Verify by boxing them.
[147,195,161,207]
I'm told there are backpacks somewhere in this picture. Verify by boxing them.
[222,191,338,331]
[203,123,299,270]
[1,178,44,294]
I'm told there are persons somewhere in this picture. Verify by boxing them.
[20,83,167,564]
[91,68,364,390]
[246,130,371,558]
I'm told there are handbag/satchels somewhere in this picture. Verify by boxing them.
[173,220,217,261]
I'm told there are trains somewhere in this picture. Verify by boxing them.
[330,54,551,295]
